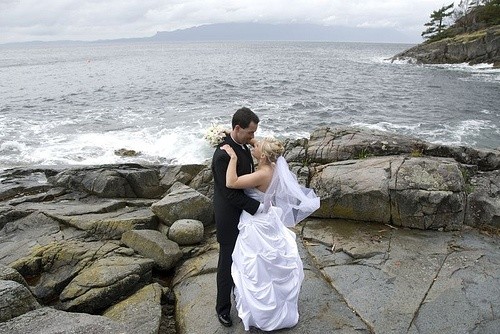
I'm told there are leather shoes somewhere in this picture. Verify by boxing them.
[217,312,232,327]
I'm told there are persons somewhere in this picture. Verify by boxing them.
[209,128,304,330]
[211,106,276,328]
